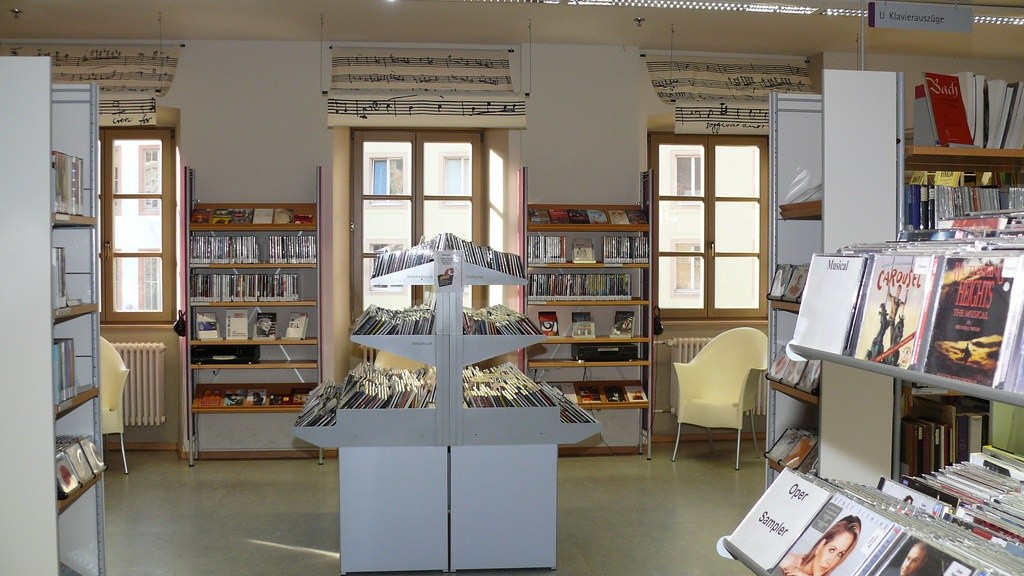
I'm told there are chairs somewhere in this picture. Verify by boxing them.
[99,336,129,475]
[670,326,768,472]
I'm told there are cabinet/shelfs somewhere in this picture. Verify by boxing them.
[819,70,1024,491]
[767,90,821,496]
[715,219,1023,576]
[448,232,602,575]
[184,166,324,467]
[292,234,449,576]
[522,165,651,460]
[1,55,107,576]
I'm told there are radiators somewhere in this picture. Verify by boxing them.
[108,342,166,428]
[662,337,768,418]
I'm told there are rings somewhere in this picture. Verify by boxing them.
[794,567,797,572]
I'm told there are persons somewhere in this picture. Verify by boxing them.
[884,283,909,345]
[779,515,861,576]
[898,541,929,576]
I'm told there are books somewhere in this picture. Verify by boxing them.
[55,434,107,493]
[725,171,1024,576]
[295,232,651,428]
[189,273,300,302]
[49,150,85,217]
[49,245,70,310]
[201,387,313,406]
[191,207,314,224]
[528,208,649,226]
[196,308,311,339]
[904,72,1023,151]
[189,235,317,264]
[51,338,78,407]
